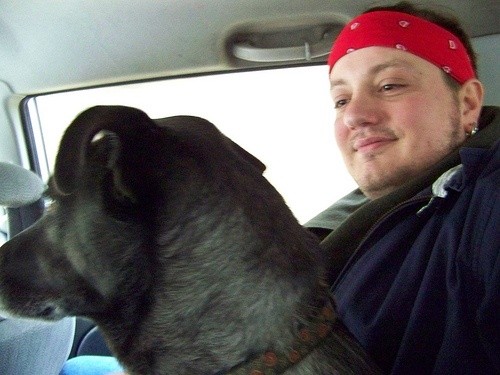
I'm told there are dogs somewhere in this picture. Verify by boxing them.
[1,105,386,375]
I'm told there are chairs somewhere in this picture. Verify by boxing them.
[0,160,76,375]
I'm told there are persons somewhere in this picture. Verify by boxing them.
[59,1,500,375]
